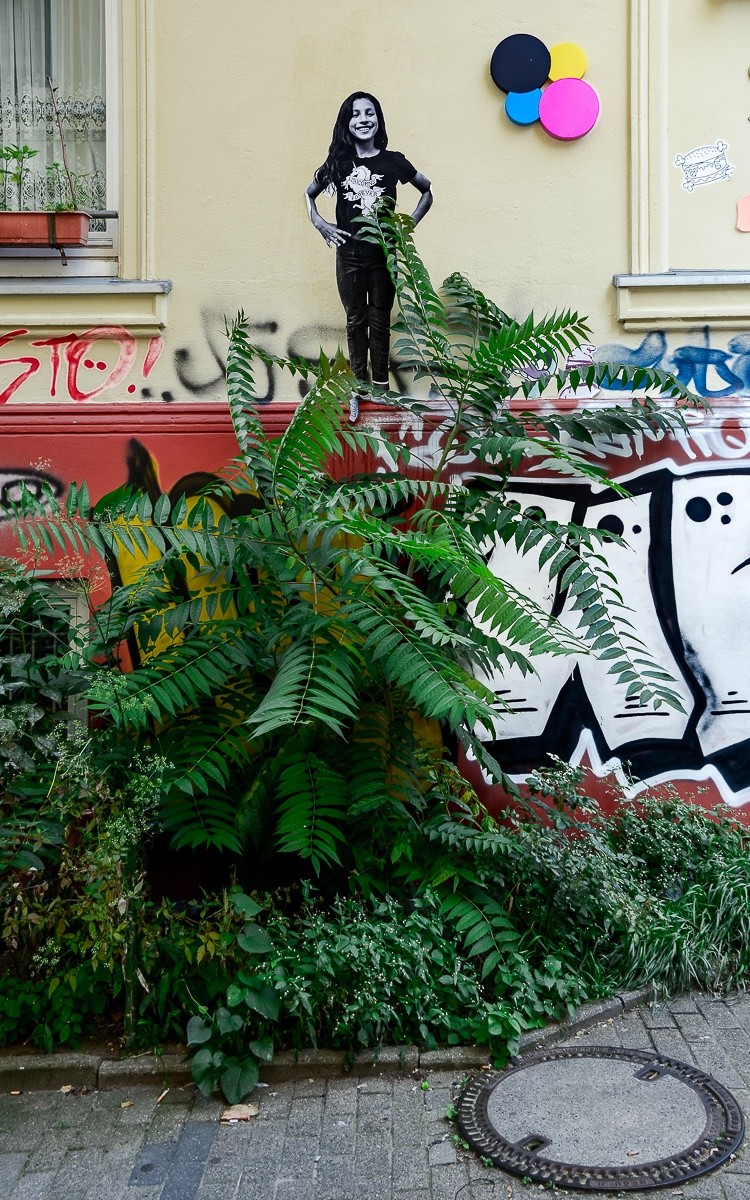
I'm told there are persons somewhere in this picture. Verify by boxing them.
[304,90,433,404]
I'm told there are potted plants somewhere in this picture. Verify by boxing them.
[0,143,94,247]
